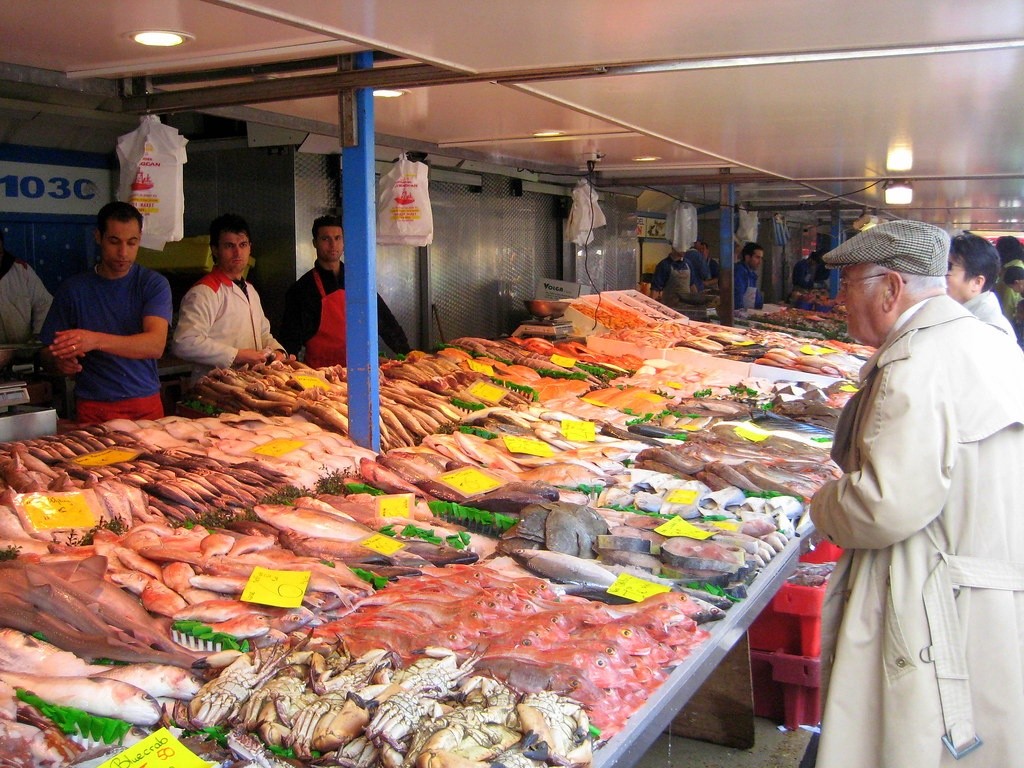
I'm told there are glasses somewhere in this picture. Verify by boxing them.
[839,274,906,295]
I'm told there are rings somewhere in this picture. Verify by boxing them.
[72,345,76,351]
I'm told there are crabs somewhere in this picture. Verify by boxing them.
[161,626,593,768]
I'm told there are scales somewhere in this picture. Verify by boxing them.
[511,300,574,339]
[810,280,826,288]
[673,291,718,323]
[0,344,49,412]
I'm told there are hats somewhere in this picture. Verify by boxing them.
[823,220,951,277]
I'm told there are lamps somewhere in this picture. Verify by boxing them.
[884,180,913,205]
[853,212,878,231]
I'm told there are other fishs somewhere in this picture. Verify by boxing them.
[0,295,880,768]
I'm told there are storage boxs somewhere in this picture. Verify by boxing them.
[134,233,257,281]
[750,540,846,731]
[536,280,590,301]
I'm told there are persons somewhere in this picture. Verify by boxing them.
[0,227,54,344]
[650,234,1024,350]
[38,201,172,427]
[278,215,411,369]
[809,219,1023,768]
[172,212,288,388]
